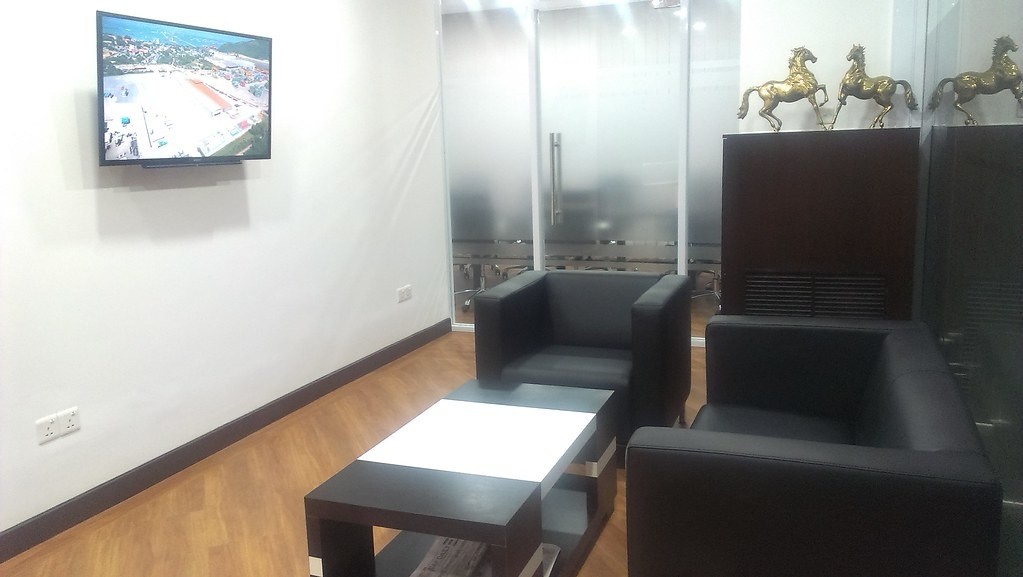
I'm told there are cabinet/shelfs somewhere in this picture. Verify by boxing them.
[721,127,921,322]
[912,125,1023,503]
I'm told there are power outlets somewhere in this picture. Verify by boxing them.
[404,284,412,299]
[397,288,406,303]
[57,406,81,436]
[33,414,59,446]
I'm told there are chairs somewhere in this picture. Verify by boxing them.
[447,169,722,316]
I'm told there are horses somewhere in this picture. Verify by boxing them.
[927,34,1023,126]
[829,42,921,130]
[736,45,830,132]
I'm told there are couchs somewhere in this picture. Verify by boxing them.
[474,269,691,468]
[625,314,1002,577]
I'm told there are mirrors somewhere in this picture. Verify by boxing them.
[920,0,1023,577]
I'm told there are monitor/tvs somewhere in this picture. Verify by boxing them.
[96,10,272,168]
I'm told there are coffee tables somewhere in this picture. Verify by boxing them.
[304,378,616,577]
